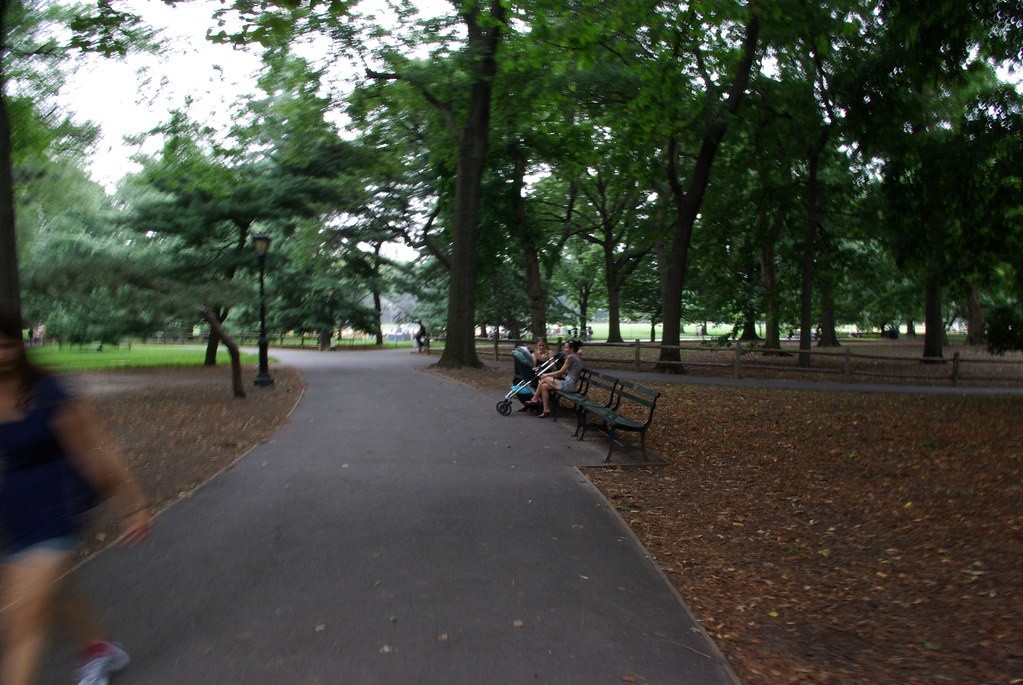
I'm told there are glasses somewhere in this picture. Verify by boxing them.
[537,337,545,340]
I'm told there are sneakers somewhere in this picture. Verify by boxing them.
[76,643,132,685]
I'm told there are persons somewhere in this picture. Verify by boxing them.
[524,338,583,417]
[413,319,427,350]
[515,337,557,412]
[487,323,594,342]
[3,296,151,683]
[28,327,41,345]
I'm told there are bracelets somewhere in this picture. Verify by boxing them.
[121,502,150,518]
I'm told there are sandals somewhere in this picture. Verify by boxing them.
[526,400,552,418]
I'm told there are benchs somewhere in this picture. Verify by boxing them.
[550,366,661,463]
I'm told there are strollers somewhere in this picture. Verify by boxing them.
[497,346,565,417]
